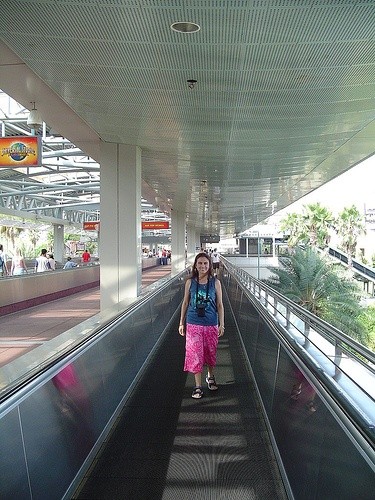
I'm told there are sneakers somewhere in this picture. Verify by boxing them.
[214,273,217,275]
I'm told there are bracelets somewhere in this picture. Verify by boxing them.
[219,325,225,329]
[179,324,184,326]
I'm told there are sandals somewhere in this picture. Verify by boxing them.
[206,374,218,389]
[192,387,203,399]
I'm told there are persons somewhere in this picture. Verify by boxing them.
[211,248,220,277]
[35,249,51,272]
[10,248,28,275]
[178,253,225,399]
[82,250,90,263]
[0,244,9,276]
[47,252,56,270]
[63,257,77,269]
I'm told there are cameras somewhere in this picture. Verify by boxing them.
[196,304,207,317]
